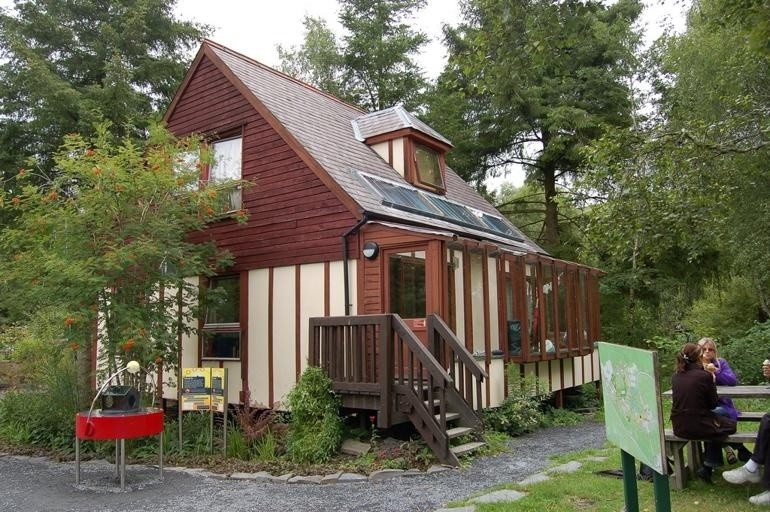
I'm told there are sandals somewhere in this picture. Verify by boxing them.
[726,450,737,464]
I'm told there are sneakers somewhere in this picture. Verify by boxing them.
[738,452,752,463]
[749,491,770,505]
[722,466,761,484]
[698,467,714,486]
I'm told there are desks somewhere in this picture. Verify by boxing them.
[661,385,770,490]
[76,408,164,490]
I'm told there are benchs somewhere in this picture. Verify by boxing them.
[663,409,767,490]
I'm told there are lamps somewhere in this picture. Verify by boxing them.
[362,240,378,260]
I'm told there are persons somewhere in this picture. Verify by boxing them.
[697,337,739,463]
[671,343,752,485]
[722,358,770,504]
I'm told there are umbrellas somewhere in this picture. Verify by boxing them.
[531,299,539,351]
